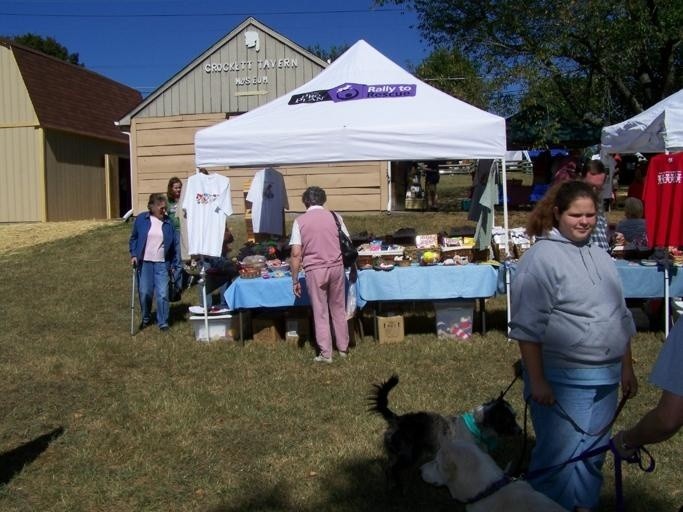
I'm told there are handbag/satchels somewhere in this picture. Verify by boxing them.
[330,209,357,267]
[167,269,181,302]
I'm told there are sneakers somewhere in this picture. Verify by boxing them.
[312,353,332,364]
[139,322,147,330]
[338,350,348,357]
[161,327,168,333]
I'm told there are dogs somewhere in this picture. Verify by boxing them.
[419,415,570,512]
[365,372,523,512]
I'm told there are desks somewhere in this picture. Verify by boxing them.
[235,261,497,348]
[504,254,681,298]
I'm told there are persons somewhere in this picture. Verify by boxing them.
[613,315,682,460]
[578,160,609,252]
[420,160,440,210]
[163,177,184,293]
[509,178,638,510]
[129,192,178,330]
[191,226,234,308]
[614,196,647,243]
[614,230,648,247]
[288,187,352,364]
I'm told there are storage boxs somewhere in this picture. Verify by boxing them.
[187,315,240,344]
[251,317,285,347]
[431,299,476,341]
[285,317,310,339]
[373,313,404,345]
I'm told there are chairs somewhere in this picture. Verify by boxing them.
[461,178,534,213]
